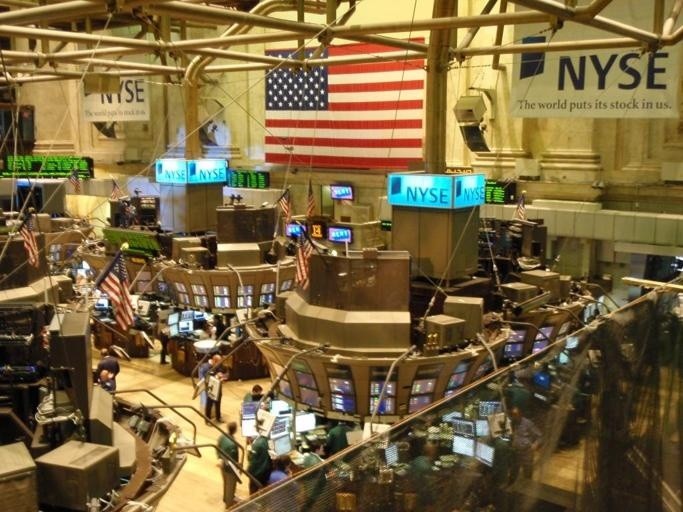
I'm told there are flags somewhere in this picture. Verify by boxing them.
[109,180,120,200]
[258,36,427,170]
[93,253,135,331]
[305,183,316,220]
[516,196,526,220]
[68,173,81,194]
[16,213,39,270]
[277,188,291,223]
[292,229,313,292]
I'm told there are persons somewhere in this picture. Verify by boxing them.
[118,199,137,228]
[411,315,427,354]
[509,301,663,444]
[201,354,229,426]
[256,302,273,323]
[157,328,170,365]
[97,370,116,393]
[91,347,120,383]
[213,312,227,342]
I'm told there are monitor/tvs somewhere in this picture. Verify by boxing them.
[407,378,436,414]
[273,434,292,454]
[442,346,497,407]
[583,294,606,323]
[451,435,475,458]
[330,184,354,201]
[298,385,321,409]
[371,422,393,435]
[450,417,476,438]
[329,392,357,415]
[328,376,356,395]
[478,400,503,417]
[272,362,294,399]
[369,379,397,397]
[474,441,495,467]
[475,419,492,438]
[369,397,397,416]
[241,399,316,439]
[502,319,580,389]
[384,445,400,468]
[95,279,293,339]
[442,410,465,424]
[292,369,319,390]
[327,226,353,244]
[285,223,308,237]
